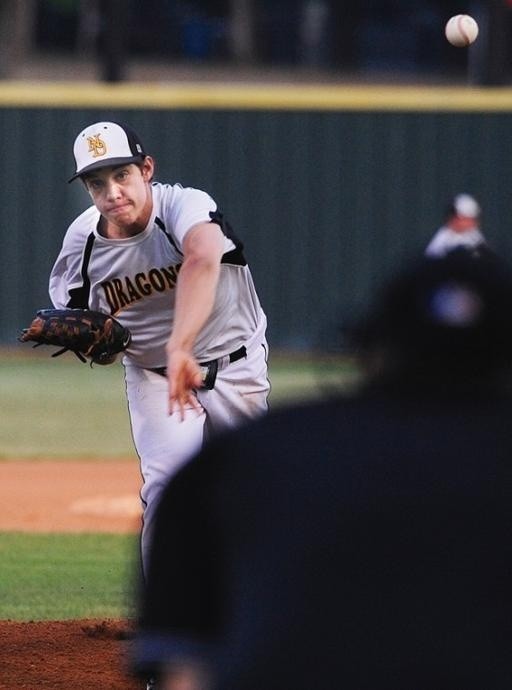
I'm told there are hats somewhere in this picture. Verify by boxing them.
[66,120,145,184]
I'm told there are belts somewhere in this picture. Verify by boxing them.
[146,345,245,389]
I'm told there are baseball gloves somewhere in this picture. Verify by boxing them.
[18,309,133,361]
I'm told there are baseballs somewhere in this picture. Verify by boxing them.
[444,14,479,48]
